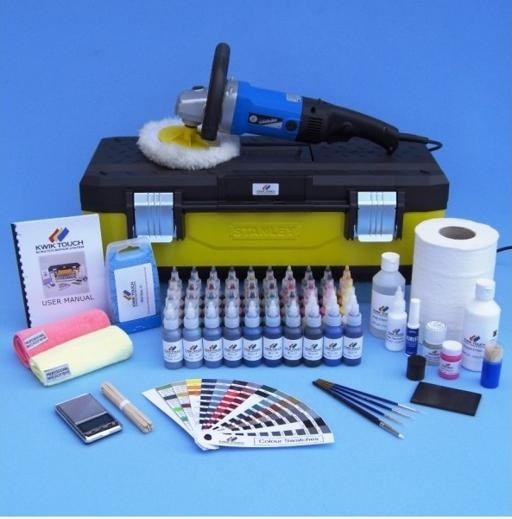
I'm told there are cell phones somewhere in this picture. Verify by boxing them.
[410,382,481,416]
[55,392,123,444]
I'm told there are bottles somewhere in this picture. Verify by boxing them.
[162,266,363,370]
[384,284,464,380]
[369,251,406,340]
[460,279,504,389]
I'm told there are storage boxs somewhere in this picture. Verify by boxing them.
[79,135,450,281]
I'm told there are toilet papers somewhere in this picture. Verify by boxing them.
[409,217,500,341]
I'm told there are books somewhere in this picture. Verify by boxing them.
[10,213,105,329]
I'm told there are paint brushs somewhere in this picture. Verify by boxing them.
[313,381,421,439]
